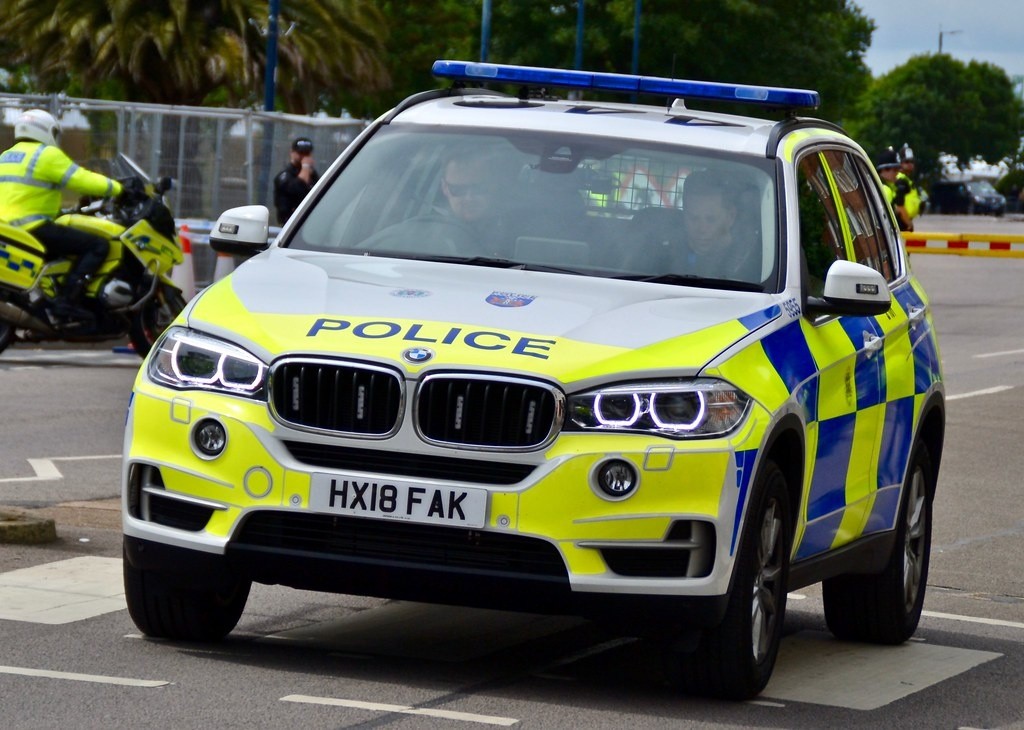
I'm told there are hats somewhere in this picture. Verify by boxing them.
[876,146,901,170]
[292,137,313,152]
[900,143,916,163]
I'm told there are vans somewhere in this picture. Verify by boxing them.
[929,180,1006,217]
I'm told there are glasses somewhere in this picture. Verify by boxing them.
[442,174,489,197]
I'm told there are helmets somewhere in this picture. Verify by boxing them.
[14,109,62,149]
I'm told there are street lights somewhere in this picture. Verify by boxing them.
[938,30,961,52]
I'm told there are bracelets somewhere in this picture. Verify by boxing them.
[302,163,311,169]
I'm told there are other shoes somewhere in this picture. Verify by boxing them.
[78,293,118,331]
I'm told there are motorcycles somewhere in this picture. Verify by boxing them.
[0,153,188,359]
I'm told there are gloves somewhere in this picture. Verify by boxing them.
[112,187,139,208]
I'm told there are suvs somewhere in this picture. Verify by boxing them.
[119,60,946,703]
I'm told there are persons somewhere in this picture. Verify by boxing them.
[642,168,760,284]
[0,108,137,321]
[273,135,321,224]
[432,149,556,256]
[876,148,922,232]
